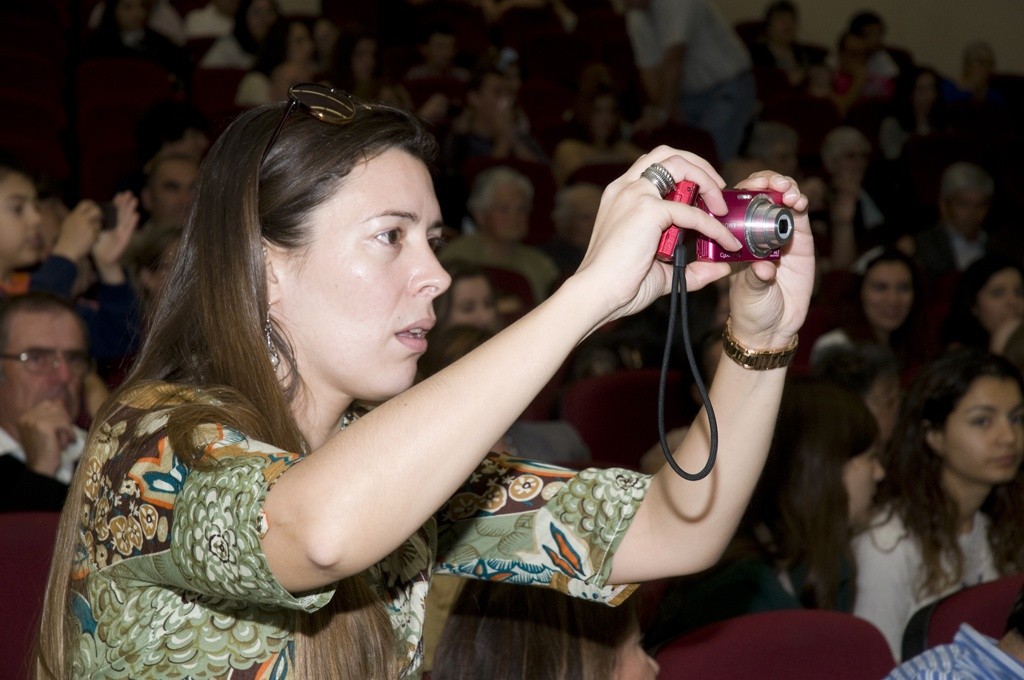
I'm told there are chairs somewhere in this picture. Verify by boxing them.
[1,512,67,679]
[654,612,895,680]
[471,153,865,459]
[903,577,1024,664]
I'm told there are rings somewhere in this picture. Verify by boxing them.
[640,163,674,196]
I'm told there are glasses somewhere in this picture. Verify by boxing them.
[260,80,372,168]
[0,348,90,379]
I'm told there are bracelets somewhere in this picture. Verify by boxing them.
[722,314,798,371]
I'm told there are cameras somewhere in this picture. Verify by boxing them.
[695,188,794,263]
[656,180,700,264]
[98,204,116,230]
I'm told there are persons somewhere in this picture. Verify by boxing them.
[1,0,1023,679]
[35,101,816,680]
